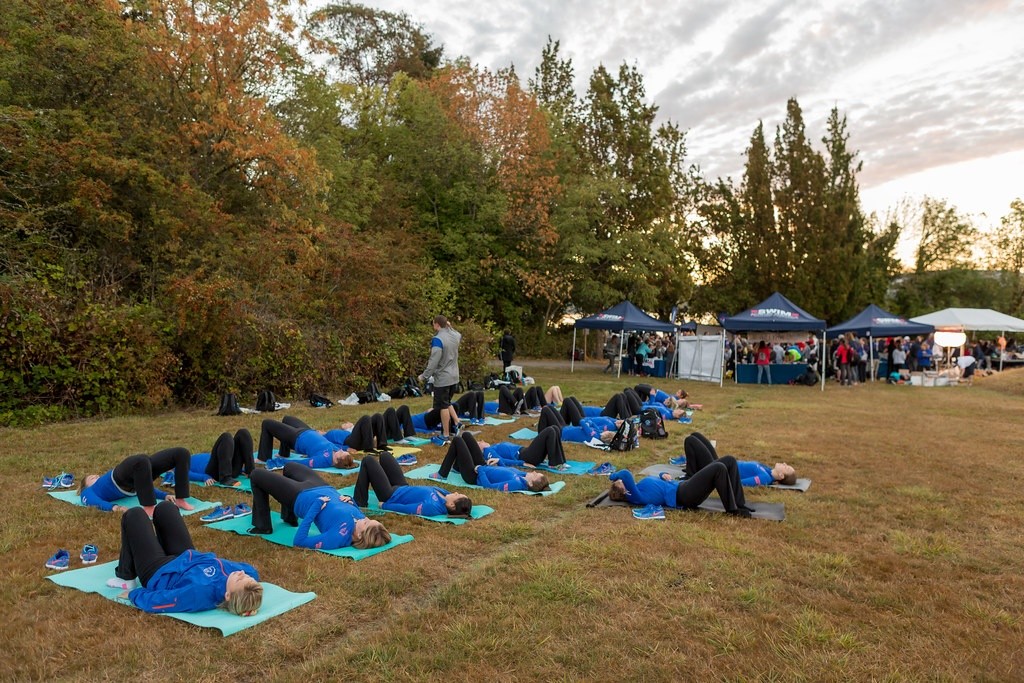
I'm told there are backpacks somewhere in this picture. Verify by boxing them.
[759,352,765,360]
[609,419,638,451]
[218,370,535,415]
[640,408,668,439]
[909,342,918,357]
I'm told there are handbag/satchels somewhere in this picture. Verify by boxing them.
[498,352,502,360]
[860,346,868,361]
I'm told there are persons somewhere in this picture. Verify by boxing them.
[451,390,487,425]
[77,447,195,518]
[475,425,572,471]
[538,383,694,441]
[607,432,797,520]
[400,409,460,436]
[247,462,392,550]
[104,500,261,616]
[416,316,462,439]
[352,452,474,521]
[171,428,256,487]
[253,405,415,470]
[428,431,553,492]
[485,384,565,416]
[604,333,1024,388]
[499,329,516,371]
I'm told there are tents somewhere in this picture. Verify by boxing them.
[724,291,828,390]
[569,299,680,377]
[908,308,1024,380]
[826,304,938,383]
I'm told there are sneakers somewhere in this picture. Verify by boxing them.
[159,471,175,487]
[478,419,486,425]
[453,422,465,438]
[633,418,641,423]
[469,418,476,425]
[80,544,98,564]
[669,456,687,466]
[200,505,234,521]
[678,416,692,424]
[438,435,451,444]
[46,549,70,569]
[530,406,540,412]
[632,504,665,519]
[234,503,252,517]
[264,456,287,471]
[42,473,75,490]
[431,437,445,447]
[587,463,616,475]
[396,454,417,465]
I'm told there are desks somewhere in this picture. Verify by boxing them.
[737,363,807,383]
[621,354,665,377]
